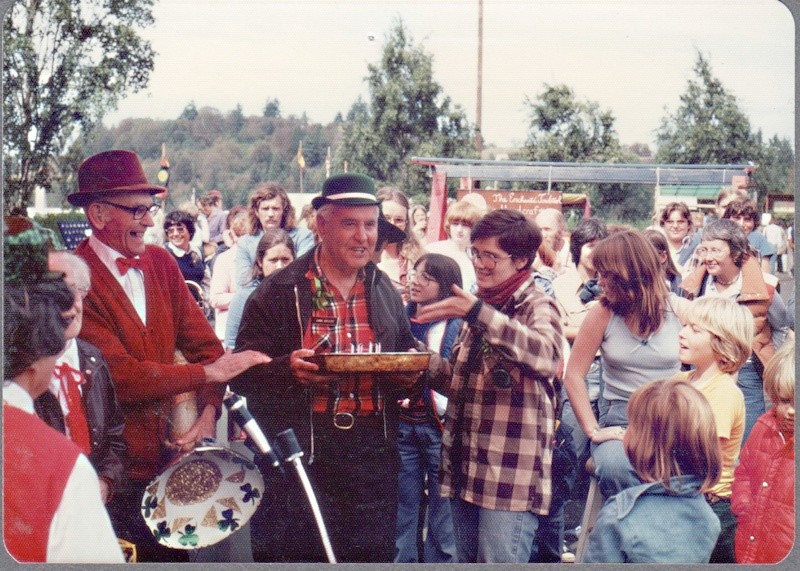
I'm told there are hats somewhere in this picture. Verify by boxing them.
[311,174,381,210]
[67,150,165,207]
[378,209,407,244]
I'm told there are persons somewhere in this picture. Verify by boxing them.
[402,251,465,565]
[31,253,138,564]
[414,209,563,564]
[1,286,128,565]
[672,294,747,562]
[69,151,270,561]
[565,229,693,497]
[585,379,723,565]
[231,173,423,562]
[147,161,795,348]
[732,342,796,563]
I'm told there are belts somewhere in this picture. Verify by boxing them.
[312,413,397,431]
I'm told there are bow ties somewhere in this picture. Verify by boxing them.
[115,257,144,276]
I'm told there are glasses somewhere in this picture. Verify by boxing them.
[696,247,729,256]
[464,246,520,269]
[385,217,405,224]
[409,270,438,287]
[166,227,185,233]
[94,201,162,220]
[664,220,687,226]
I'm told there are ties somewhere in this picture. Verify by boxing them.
[61,363,92,456]
